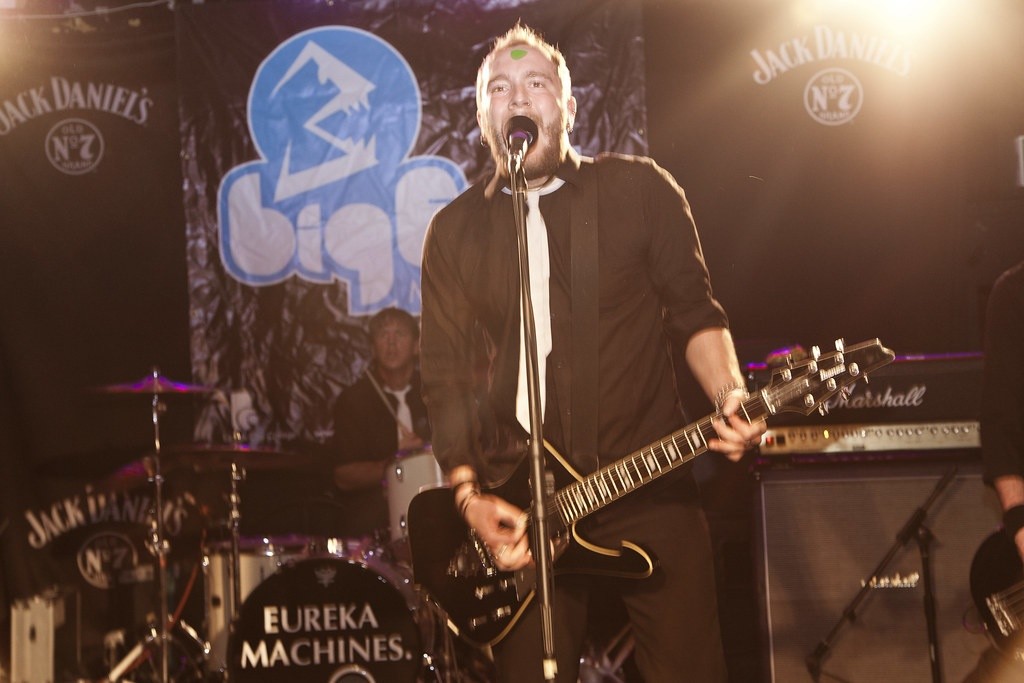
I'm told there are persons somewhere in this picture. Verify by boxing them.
[416,24,767,683]
[332,307,430,537]
[978,260,1024,562]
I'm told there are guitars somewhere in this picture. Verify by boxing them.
[972,524,1023,651]
[404,333,898,648]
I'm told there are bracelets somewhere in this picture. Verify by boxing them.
[1003,504,1024,544]
[714,381,747,409]
[450,480,479,517]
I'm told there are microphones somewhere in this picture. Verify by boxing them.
[503,116,538,173]
[803,653,822,683]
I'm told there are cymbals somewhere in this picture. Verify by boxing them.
[96,378,214,396]
[167,444,287,462]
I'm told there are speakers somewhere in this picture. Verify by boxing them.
[747,455,1006,683]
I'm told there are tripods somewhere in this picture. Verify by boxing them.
[101,390,231,683]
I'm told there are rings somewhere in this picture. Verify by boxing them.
[746,440,751,447]
[497,544,507,557]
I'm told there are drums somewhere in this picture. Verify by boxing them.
[225,545,463,683]
[195,538,382,683]
